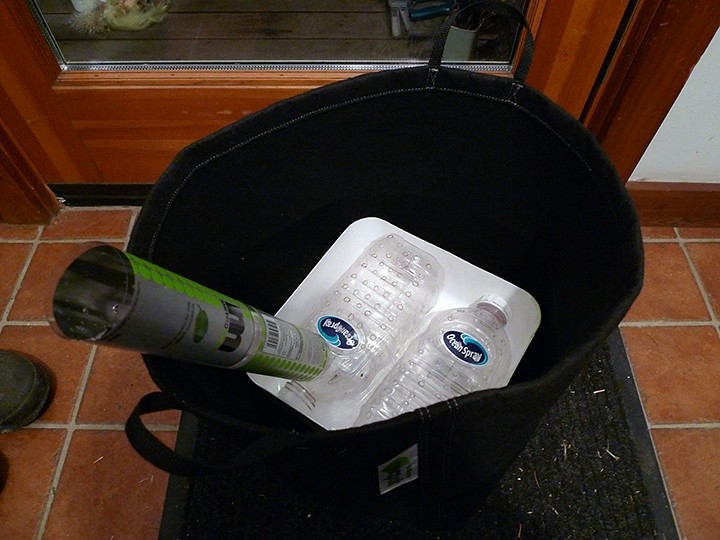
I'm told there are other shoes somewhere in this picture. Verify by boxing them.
[0,349,51,427]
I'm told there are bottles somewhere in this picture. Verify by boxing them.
[278,233,445,416]
[353,293,512,427]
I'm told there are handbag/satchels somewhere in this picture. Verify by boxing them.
[126,1,644,539]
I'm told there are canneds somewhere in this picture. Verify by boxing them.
[49,243,329,382]
